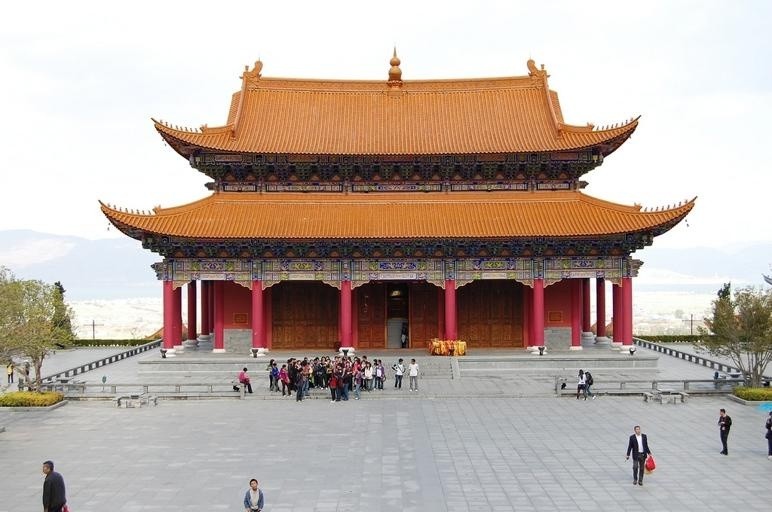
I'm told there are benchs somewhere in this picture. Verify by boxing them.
[643,389,689,404]
[112,393,157,407]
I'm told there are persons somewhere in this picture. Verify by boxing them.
[427,338,467,355]
[7,360,14,383]
[718,409,732,455]
[626,426,652,485]
[408,359,419,392]
[583,372,596,400]
[394,359,405,388]
[25,360,30,382]
[576,370,588,401]
[765,412,772,458]
[239,368,253,393]
[42,460,66,512]
[266,355,385,401]
[244,479,264,512]
